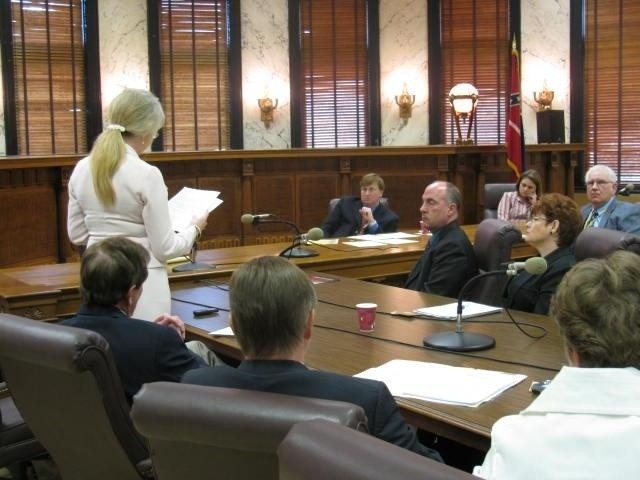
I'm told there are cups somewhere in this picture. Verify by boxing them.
[419,220,429,234]
[354,302,377,333]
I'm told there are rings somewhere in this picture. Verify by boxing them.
[366,212,369,216]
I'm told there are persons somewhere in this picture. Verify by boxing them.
[496,168,543,227]
[319,172,400,239]
[579,165,640,235]
[182,255,446,466]
[502,192,586,317]
[404,179,480,302]
[64,87,209,324]
[56,236,228,406]
[470,249,638,480]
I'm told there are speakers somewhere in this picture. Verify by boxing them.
[537,110,565,144]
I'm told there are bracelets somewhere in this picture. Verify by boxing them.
[193,224,203,240]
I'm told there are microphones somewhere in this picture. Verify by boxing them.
[500,257,548,275]
[241,213,274,225]
[618,184,635,196]
[294,227,324,240]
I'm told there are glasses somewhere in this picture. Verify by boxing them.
[150,132,159,139]
[588,179,612,185]
[531,216,549,222]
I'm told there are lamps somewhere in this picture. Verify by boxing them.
[394,81,416,131]
[448,83,479,145]
[257,82,279,129]
[533,80,555,111]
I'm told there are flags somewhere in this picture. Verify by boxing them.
[505,48,523,183]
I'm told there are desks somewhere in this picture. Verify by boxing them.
[0,220,581,451]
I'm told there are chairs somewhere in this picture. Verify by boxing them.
[329,197,389,216]
[483,183,518,219]
[277,418,485,480]
[571,227,640,264]
[127,381,368,480]
[0,313,157,480]
